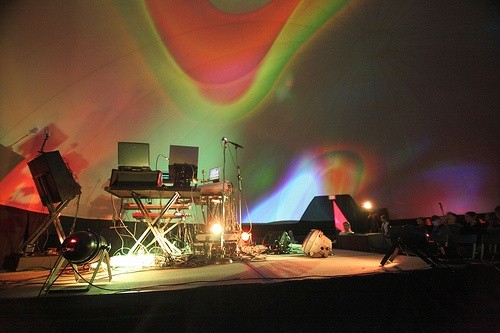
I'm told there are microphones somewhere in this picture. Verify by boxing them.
[274,239,281,245]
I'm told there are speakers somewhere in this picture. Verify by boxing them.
[302,229,333,257]
[27,151,77,205]
[195,232,246,249]
[262,231,290,252]
[338,232,385,251]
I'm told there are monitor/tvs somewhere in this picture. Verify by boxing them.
[208,167,220,184]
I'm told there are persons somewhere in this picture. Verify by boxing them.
[415,205,500,262]
[333,222,355,244]
[380,215,392,251]
